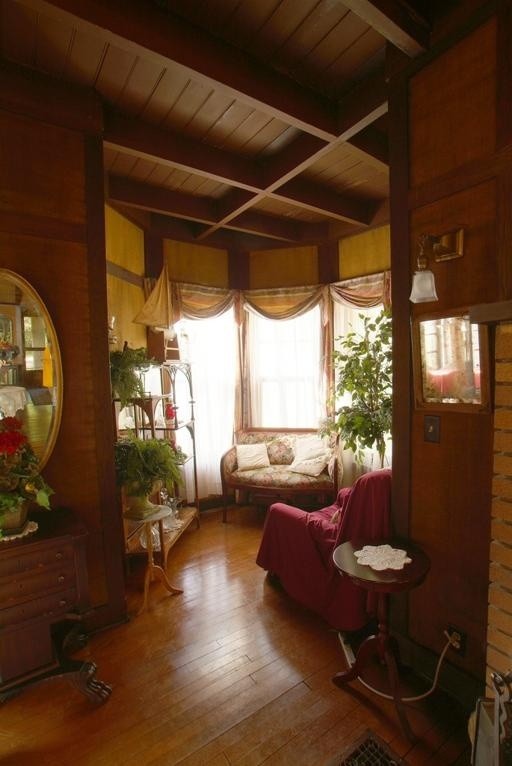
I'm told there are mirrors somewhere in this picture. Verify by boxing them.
[406,303,498,418]
[0,267,65,491]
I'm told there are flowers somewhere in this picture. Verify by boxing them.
[0,342,19,362]
[0,406,58,518]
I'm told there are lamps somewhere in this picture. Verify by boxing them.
[404,223,470,305]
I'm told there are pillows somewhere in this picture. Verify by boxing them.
[286,445,336,477]
[232,440,269,473]
[265,436,297,467]
[290,435,329,462]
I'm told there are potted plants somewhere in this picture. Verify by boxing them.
[111,423,189,522]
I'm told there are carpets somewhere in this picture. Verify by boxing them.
[322,725,415,766]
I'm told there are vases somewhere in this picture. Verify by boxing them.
[0,493,31,539]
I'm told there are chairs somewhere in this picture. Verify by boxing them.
[249,465,394,648]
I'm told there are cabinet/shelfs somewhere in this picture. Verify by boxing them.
[1,502,115,719]
[108,357,203,573]
[142,323,180,363]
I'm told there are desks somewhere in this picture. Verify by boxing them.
[121,503,185,619]
[316,535,434,753]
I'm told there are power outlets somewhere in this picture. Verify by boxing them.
[445,622,469,659]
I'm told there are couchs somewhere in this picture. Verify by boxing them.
[218,425,343,526]
